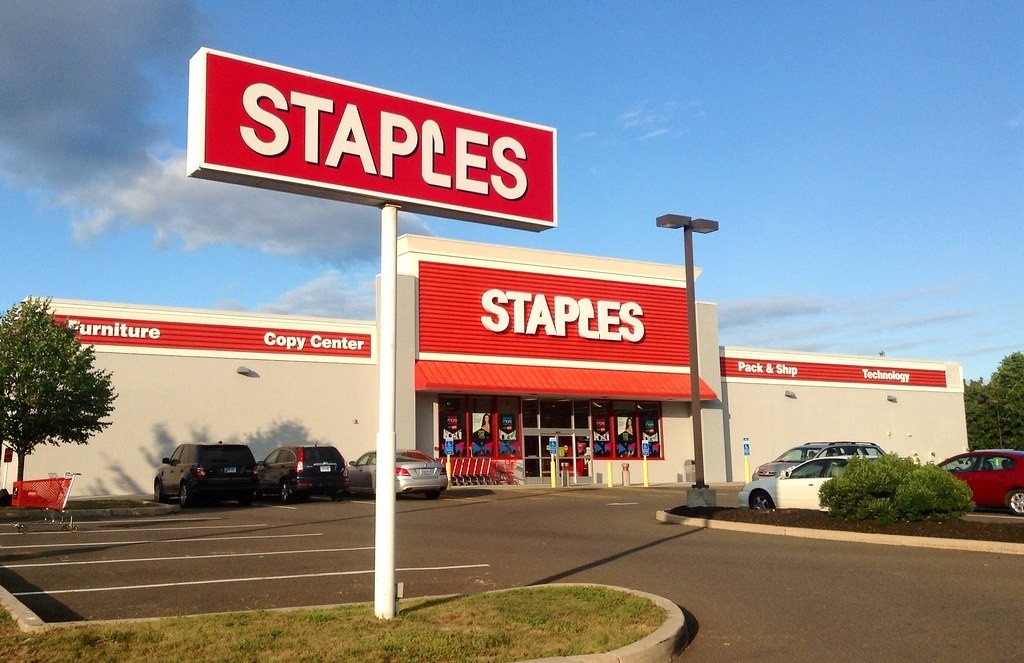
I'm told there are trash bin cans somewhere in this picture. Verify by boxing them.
[683,459,697,481]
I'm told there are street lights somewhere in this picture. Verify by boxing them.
[656,214,719,507]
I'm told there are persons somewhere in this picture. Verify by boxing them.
[624,417,633,437]
[482,414,490,432]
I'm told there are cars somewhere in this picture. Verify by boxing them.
[738,455,880,516]
[937,448,1024,516]
[348,449,448,501]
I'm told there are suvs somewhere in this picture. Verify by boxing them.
[261,444,349,505]
[752,441,888,484]
[154,441,262,510]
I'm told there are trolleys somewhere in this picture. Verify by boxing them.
[11,471,82,535]
